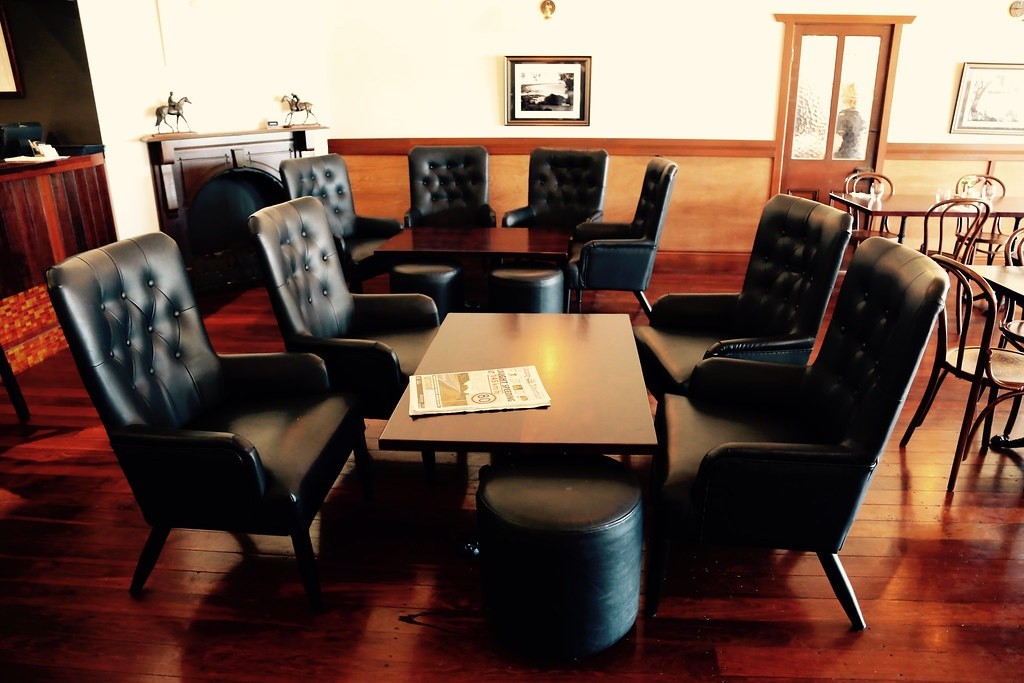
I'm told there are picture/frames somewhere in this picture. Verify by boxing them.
[0,7,21,97]
[504,54,592,127]
[949,61,1024,135]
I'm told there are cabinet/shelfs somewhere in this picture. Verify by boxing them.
[139,123,330,244]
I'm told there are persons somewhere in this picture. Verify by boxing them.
[168,91,178,110]
[291,93,300,111]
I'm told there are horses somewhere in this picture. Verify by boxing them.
[155,97,192,132]
[282,95,318,125]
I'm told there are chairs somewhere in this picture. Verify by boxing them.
[404,145,496,227]
[564,156,679,320]
[642,236,951,627]
[500,147,608,227]
[279,153,404,292]
[632,194,855,402]
[45,232,371,606]
[247,194,465,490]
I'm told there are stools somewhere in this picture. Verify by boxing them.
[387,262,458,324]
[476,452,642,659]
[490,264,564,312]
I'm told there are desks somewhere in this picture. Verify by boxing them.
[377,311,659,535]
[374,223,576,318]
[964,264,1024,450]
[842,171,1024,490]
[829,192,1024,317]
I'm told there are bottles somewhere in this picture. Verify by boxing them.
[962,177,978,207]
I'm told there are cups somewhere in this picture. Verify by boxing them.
[871,183,884,200]
[983,184,996,201]
[937,188,951,205]
